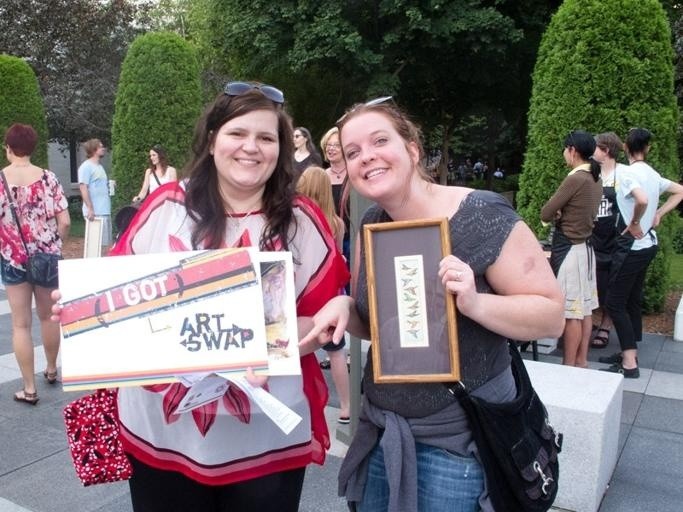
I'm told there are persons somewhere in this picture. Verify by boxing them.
[424,148,503,187]
[51,77,351,512]
[77,139,113,257]
[589,131,625,349]
[293,124,365,427]
[298,96,566,512]
[539,128,602,368]
[597,127,683,379]
[132,144,177,202]
[0,123,71,406]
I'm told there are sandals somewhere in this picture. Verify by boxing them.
[592,330,610,349]
[44,371,58,384]
[16,391,40,404]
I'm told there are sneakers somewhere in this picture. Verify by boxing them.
[599,364,640,378]
[600,353,637,364]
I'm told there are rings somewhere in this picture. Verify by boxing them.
[456,271,461,281]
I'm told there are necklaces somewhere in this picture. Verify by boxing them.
[224,206,257,232]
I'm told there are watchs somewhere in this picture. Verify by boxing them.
[632,221,640,225]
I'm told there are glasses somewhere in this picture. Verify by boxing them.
[335,96,404,133]
[224,81,285,104]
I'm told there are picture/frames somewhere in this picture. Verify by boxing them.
[363,217,461,384]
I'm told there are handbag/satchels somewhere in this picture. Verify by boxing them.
[26,253,63,287]
[64,391,132,487]
[460,345,562,511]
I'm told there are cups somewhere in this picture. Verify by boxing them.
[108,180,118,197]
